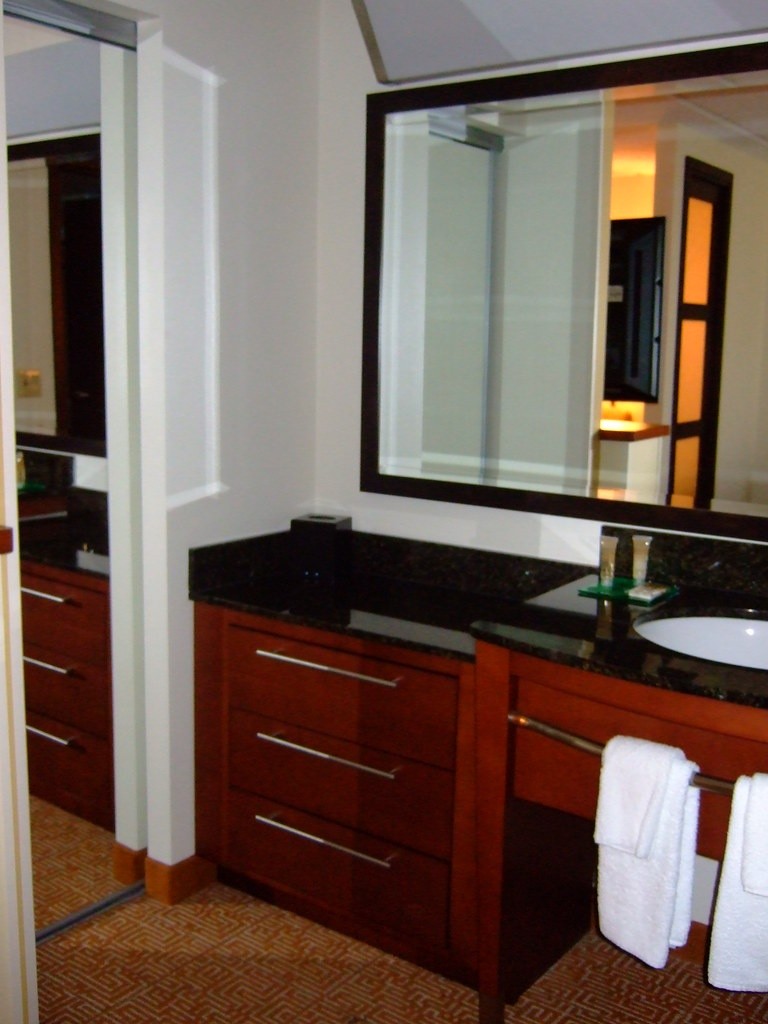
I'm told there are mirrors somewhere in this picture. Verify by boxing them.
[3,3,147,942]
[360,41,768,543]
[6,133,106,458]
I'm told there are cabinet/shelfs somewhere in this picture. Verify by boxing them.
[193,601,475,986]
[20,573,115,835]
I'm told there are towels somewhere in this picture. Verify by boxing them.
[707,772,767,992]
[593,733,701,971]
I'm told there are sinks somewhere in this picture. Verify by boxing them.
[633,606,768,672]
